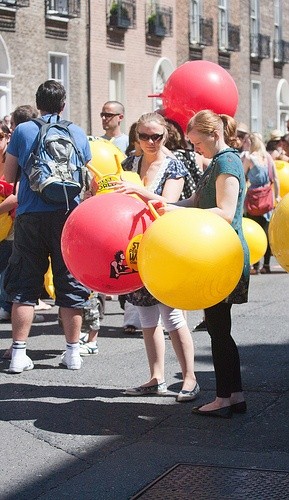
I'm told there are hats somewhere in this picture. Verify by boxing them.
[270,130,281,140]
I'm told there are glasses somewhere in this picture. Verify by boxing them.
[137,131,165,142]
[0,132,8,138]
[100,113,120,117]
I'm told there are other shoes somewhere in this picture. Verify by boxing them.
[192,404,232,418]
[232,401,247,414]
[34,299,52,310]
[0,307,11,320]
[126,382,167,395]
[250,269,257,275]
[33,315,44,323]
[260,266,271,274]
[177,383,200,400]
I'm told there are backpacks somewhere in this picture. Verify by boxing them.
[23,118,88,202]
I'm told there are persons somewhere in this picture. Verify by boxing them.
[235,120,289,275]
[5,80,92,373]
[0,105,52,323]
[148,109,250,419]
[58,101,213,356]
[84,113,200,402]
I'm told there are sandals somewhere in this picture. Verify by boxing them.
[123,325,136,335]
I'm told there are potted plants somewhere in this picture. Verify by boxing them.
[148,13,166,37]
[108,4,131,28]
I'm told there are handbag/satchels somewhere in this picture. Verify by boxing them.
[244,183,273,216]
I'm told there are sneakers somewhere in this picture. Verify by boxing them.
[62,356,81,369]
[10,355,34,372]
[64,343,99,356]
